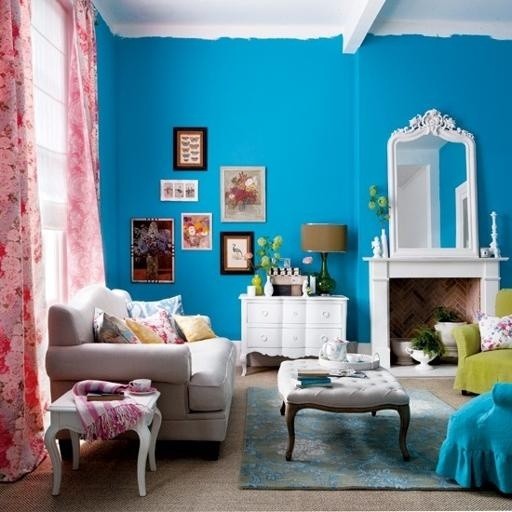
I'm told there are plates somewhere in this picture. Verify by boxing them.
[128,391,155,396]
[319,353,380,370]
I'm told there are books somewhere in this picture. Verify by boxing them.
[296,369,331,387]
[86,392,122,401]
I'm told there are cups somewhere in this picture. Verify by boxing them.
[480,248,491,257]
[129,379,151,391]
[247,286,256,297]
[347,354,361,362]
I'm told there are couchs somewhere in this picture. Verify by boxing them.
[436,382,512,494]
[44,283,237,462]
[452,289,512,395]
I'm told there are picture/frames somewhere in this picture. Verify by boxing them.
[219,167,266,223]
[160,179,199,201]
[172,126,208,171]
[218,231,256,275]
[180,212,213,251]
[129,217,175,285]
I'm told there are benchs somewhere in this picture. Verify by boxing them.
[277,358,412,463]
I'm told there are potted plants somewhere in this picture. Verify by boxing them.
[430,304,469,351]
[406,324,445,374]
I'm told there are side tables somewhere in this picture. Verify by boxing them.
[42,380,161,498]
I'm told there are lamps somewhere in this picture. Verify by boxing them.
[301,222,348,295]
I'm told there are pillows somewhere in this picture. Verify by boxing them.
[125,318,165,344]
[473,309,512,352]
[92,306,142,345]
[126,294,185,338]
[172,313,216,342]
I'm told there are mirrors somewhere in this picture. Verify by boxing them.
[386,108,480,260]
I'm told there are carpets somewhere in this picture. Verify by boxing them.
[238,385,472,491]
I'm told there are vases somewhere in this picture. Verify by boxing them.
[252,272,264,296]
[381,228,390,259]
[264,271,274,296]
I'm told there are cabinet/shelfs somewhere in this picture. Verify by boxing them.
[360,255,509,370]
[238,290,350,378]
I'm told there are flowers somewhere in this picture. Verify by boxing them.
[258,233,286,275]
[368,184,391,229]
[244,252,259,273]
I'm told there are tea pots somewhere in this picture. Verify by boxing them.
[321,336,350,362]
